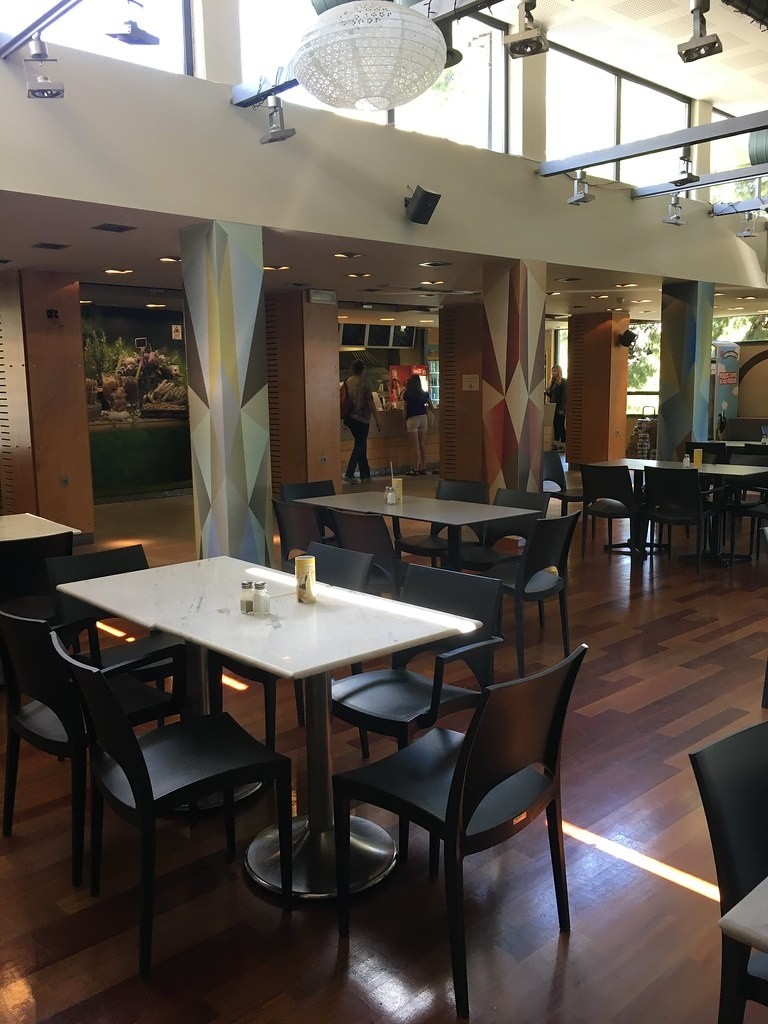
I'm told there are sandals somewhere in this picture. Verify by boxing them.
[420,469,428,476]
[404,468,419,476]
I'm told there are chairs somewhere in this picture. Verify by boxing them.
[0,444,768,1024]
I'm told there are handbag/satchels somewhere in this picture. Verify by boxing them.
[338,376,352,420]
[549,379,556,404]
[425,391,436,428]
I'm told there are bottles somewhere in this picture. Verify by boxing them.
[387,487,395,504]
[430,362,439,399]
[683,454,690,467]
[391,369,401,398]
[240,579,255,614]
[761,436,767,445]
[384,487,390,504]
[252,581,270,616]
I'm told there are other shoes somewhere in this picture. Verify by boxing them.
[558,446,566,453]
[362,476,374,484]
[551,444,558,450]
[344,475,359,486]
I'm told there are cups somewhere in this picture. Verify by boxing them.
[295,555,316,604]
[392,479,402,503]
[694,449,702,469]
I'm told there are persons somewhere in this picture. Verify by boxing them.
[402,375,436,476]
[545,365,567,455]
[341,359,381,484]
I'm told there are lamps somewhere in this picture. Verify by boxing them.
[502,0,549,59]
[404,184,441,225]
[736,212,758,238]
[259,93,296,143]
[567,167,595,206]
[677,0,723,63]
[22,31,65,99]
[104,0,160,45]
[618,330,637,348]
[662,193,688,227]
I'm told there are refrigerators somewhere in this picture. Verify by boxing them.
[409,364,428,406]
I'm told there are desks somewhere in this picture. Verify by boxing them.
[0,510,81,547]
[55,556,482,902]
[293,491,543,571]
[585,457,768,566]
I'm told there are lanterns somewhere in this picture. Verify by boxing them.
[285,1,447,113]
